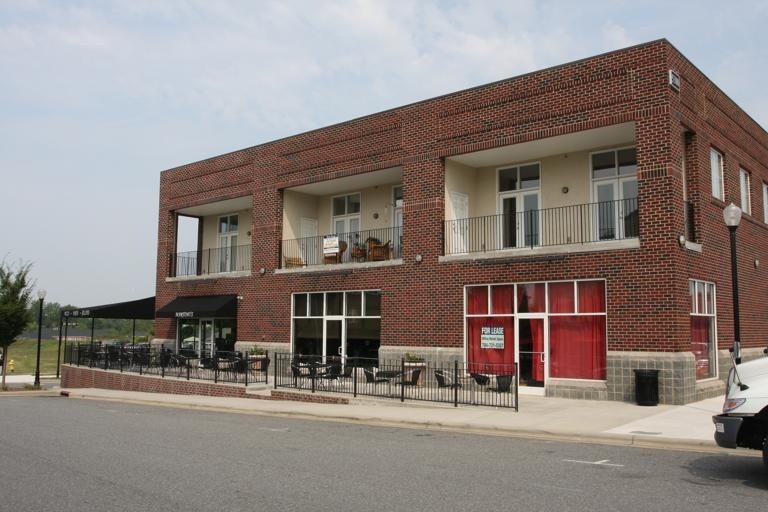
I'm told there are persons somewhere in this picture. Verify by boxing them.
[0,351,4,374]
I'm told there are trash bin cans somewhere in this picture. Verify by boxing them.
[633,368,661,406]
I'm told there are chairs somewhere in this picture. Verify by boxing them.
[66,341,270,385]
[321,235,394,265]
[291,361,514,407]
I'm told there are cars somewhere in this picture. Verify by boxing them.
[711,339,768,478]
[73,337,150,361]
[0,349,4,366]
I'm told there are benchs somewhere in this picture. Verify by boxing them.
[282,255,308,267]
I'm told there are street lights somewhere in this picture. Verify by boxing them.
[723,203,743,362]
[34,288,47,386]
